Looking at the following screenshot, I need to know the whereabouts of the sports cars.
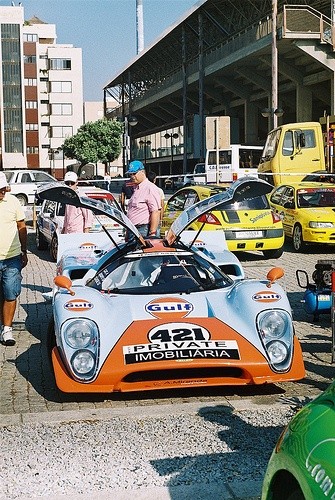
[33,177,305,393]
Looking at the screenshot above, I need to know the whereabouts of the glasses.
[65,182,75,185]
[130,172,138,175]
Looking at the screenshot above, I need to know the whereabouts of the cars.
[34,185,126,263]
[266,182,334,253]
[160,184,285,258]
[152,162,205,191]
[261,378,334,500]
[3,170,63,207]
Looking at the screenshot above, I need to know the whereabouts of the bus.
[204,145,294,184]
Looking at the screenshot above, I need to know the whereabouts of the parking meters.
[152,148,166,188]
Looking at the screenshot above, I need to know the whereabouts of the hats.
[64,171,78,183]
[125,161,144,174]
[0,171,9,189]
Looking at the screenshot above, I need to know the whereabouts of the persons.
[42,171,93,302]
[121,161,159,242]
[0,172,28,345]
[104,172,111,180]
[147,171,165,238]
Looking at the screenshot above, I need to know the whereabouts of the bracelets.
[149,231,156,235]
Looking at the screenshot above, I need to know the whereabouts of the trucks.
[258,121,335,191]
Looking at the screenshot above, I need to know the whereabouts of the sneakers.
[0,325,16,345]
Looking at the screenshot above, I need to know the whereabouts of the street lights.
[260,107,286,132]
[138,141,152,170]
[164,133,179,193]
